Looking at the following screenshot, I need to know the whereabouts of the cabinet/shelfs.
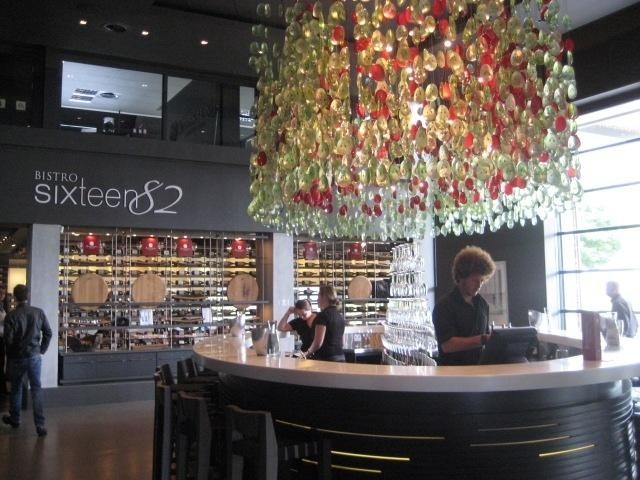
[287,233,391,335]
[59,224,272,354]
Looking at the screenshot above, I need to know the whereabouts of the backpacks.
[625,312,639,338]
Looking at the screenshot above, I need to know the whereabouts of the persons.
[431,246,496,366]
[0,285,11,395]
[606,281,638,338]
[1,284,52,437]
[277,299,319,358]
[292,285,346,362]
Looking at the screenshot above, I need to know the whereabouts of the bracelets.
[308,349,314,358]
[480,334,489,346]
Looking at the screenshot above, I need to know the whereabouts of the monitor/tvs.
[476,329,535,364]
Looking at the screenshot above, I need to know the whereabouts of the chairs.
[152,355,332,480]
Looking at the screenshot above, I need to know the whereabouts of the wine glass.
[380,241,438,367]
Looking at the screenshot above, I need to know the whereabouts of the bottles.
[294,251,393,319]
[60,246,257,346]
[608,313,619,348]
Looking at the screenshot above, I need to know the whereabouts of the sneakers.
[36,427,47,437]
[2,414,21,428]
